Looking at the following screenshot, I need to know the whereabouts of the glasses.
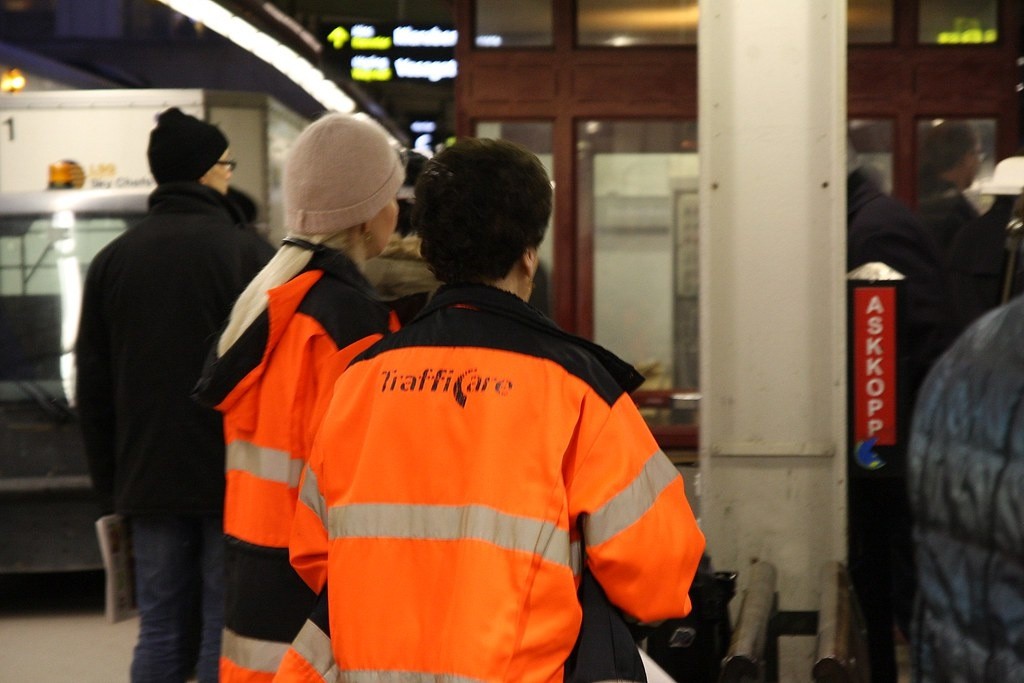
[218,159,237,178]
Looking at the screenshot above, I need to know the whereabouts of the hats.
[848,142,868,183]
[148,107,227,185]
[980,156,1024,194]
[282,114,406,234]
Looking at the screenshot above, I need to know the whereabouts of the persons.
[73,109,282,683]
[289,137,706,683]
[946,195,1024,356]
[847,138,948,683]
[895,119,979,216]
[906,295,1024,683]
[185,112,559,683]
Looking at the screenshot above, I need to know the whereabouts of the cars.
[1,185,154,574]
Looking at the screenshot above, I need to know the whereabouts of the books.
[94,513,140,623]
